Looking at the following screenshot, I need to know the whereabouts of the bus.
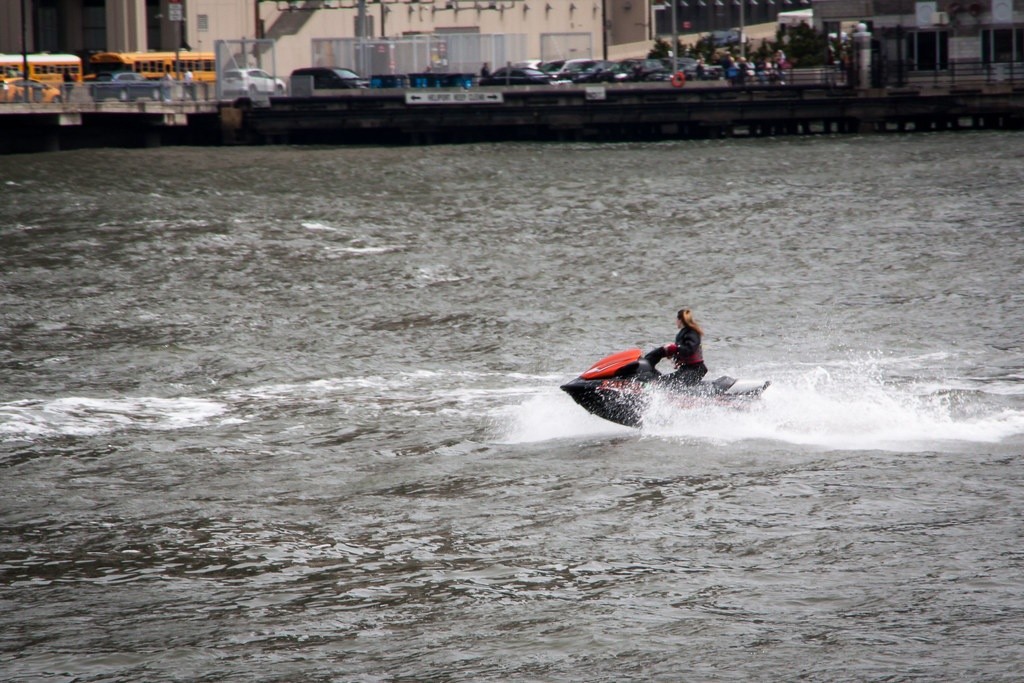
[1,53,83,94]
[87,51,218,85]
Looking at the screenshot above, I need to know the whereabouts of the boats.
[560,349,775,431]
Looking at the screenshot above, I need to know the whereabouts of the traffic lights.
[375,43,387,59]
[439,41,447,60]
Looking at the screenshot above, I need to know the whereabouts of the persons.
[6,66,17,78]
[722,50,786,85]
[481,62,490,76]
[161,71,174,101]
[181,66,196,101]
[694,52,706,81]
[63,67,75,102]
[665,308,708,387]
[828,32,851,67]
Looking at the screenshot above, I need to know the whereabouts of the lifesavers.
[671,71,686,88]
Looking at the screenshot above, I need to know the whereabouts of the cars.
[665,57,699,80]
[88,71,160,102]
[541,60,566,73]
[288,66,371,89]
[640,59,673,81]
[700,64,720,80]
[598,59,645,81]
[556,59,597,81]
[515,59,542,69]
[572,60,611,82]
[480,64,556,86]
[0,77,62,103]
[220,69,286,97]
